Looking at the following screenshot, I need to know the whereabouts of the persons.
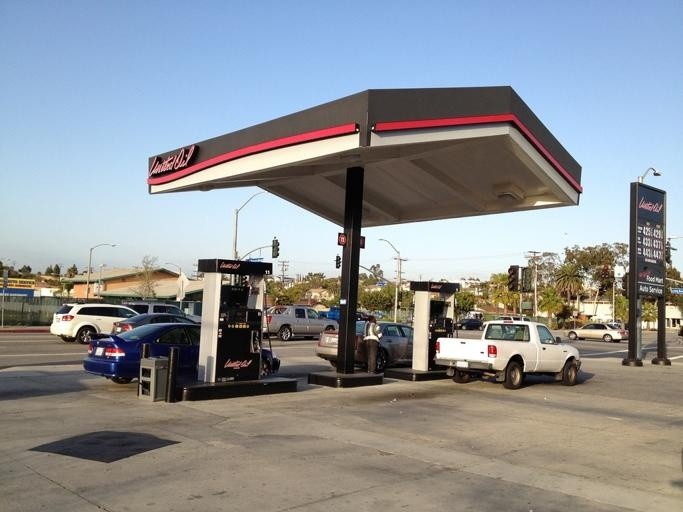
[363,315,383,373]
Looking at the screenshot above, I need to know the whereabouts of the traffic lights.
[507,266,520,293]
[335,255,341,269]
[271,238,280,258]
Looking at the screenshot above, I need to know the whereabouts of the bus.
[0,276,36,290]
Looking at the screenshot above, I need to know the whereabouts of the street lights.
[232,190,268,261]
[379,238,401,323]
[85,242,117,300]
[97,264,106,299]
[635,165,662,360]
[0,259,14,331]
[165,261,185,311]
[391,256,408,291]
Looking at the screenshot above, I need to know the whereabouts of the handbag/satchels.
[377,350,384,372]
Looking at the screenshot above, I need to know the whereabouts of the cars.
[566,321,629,344]
[46,301,281,383]
[453,311,531,331]
[261,303,415,369]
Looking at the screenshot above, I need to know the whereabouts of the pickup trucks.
[432,319,582,390]
